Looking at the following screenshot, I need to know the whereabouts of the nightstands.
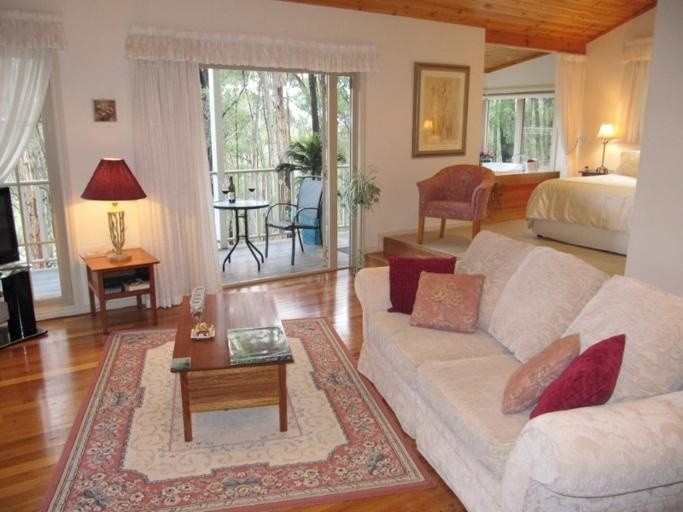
[576,167,616,178]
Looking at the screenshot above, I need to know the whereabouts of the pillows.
[406,268,487,334]
[382,251,458,318]
[498,328,584,417]
[526,332,627,420]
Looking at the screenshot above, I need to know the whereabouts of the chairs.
[412,162,497,248]
[259,174,326,267]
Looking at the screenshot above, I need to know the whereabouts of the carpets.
[34,314,439,512]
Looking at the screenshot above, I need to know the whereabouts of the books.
[225,325,292,368]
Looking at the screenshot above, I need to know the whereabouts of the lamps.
[76,153,152,263]
[592,122,618,175]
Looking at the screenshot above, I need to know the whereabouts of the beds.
[522,144,641,257]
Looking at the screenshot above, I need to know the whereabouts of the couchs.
[350,225,683,512]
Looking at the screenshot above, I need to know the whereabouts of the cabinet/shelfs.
[0,262,49,351]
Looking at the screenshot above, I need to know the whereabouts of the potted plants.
[271,130,347,247]
[342,165,382,281]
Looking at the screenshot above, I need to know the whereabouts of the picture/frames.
[409,60,473,162]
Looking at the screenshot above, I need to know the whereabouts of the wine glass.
[221,183,229,204]
[188,285,208,337]
[247,182,256,203]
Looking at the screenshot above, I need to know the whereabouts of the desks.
[212,199,270,272]
[75,245,161,338]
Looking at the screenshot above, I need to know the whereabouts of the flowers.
[476,150,496,162]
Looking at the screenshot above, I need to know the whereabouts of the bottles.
[227,176,235,203]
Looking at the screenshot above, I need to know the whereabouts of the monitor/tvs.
[0,187,20,266]
